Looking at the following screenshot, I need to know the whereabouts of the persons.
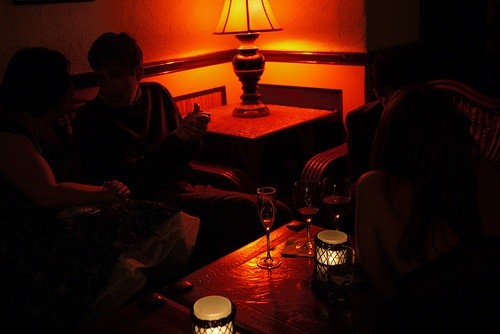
[0,32,294,334]
[346,62,500,334]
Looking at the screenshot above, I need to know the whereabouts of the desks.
[183,103,338,174]
[161,219,379,334]
[80,293,250,334]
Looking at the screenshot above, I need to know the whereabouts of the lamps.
[212,0,283,118]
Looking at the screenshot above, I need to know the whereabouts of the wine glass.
[323,177,352,232]
[295,181,320,256]
[256,188,281,268]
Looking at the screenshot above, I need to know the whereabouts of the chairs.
[301,80,500,227]
[56,96,252,195]
